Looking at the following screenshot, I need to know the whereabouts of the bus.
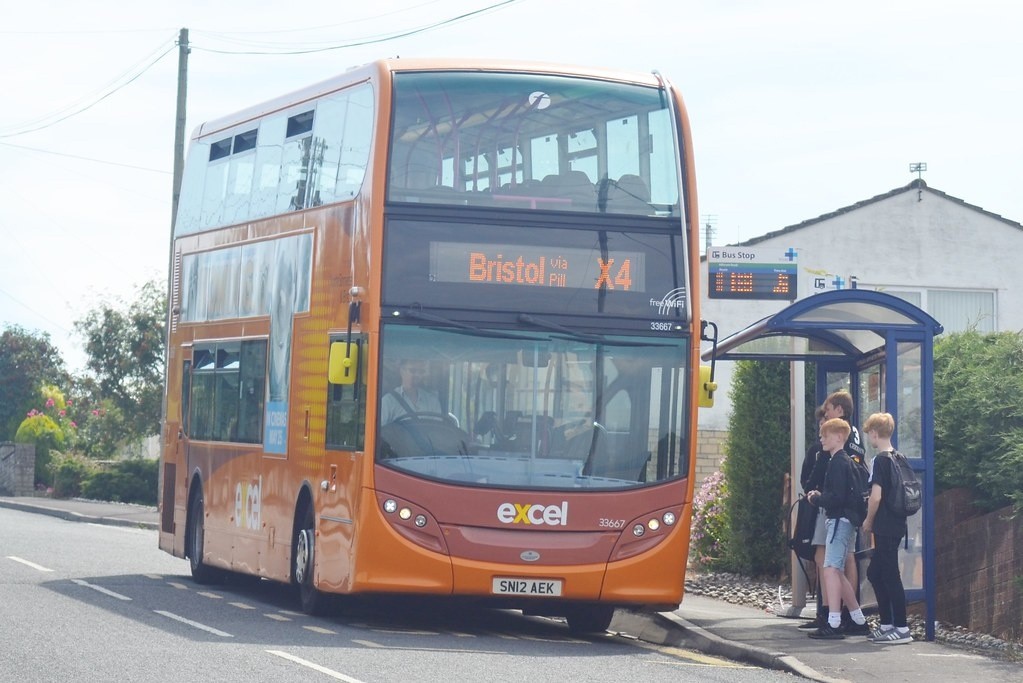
[156,56,719,637]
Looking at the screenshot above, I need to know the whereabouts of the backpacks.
[837,454,870,527]
[878,451,921,516]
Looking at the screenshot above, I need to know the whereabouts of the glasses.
[409,368,426,374]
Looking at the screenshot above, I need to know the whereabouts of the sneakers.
[867,626,894,641]
[873,627,914,644]
[842,619,869,635]
[807,622,845,639]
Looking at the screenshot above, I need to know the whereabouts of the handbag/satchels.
[794,493,818,561]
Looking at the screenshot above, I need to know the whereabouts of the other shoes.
[798,606,829,632]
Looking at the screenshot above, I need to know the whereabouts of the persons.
[381,355,442,427]
[800,390,870,639]
[864,412,914,645]
